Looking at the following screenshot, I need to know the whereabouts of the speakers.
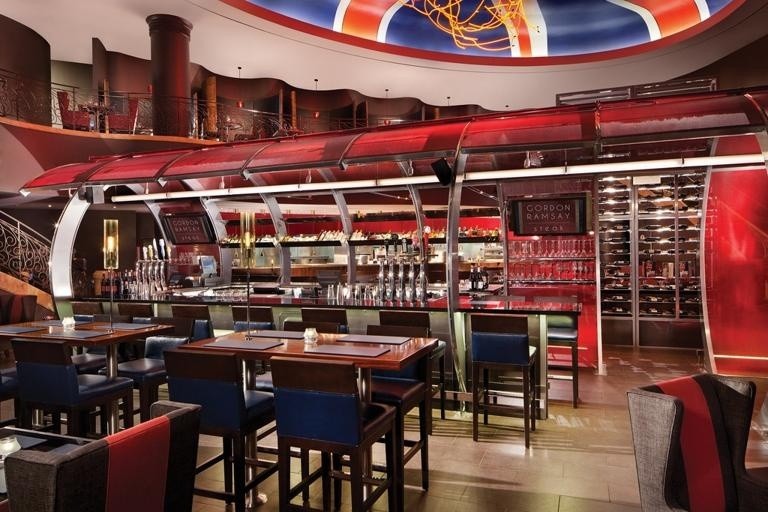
[431,158,452,186]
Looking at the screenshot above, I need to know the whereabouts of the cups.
[293,287,302,299]
[471,230,478,237]
[0,437,22,456]
[325,283,381,302]
[214,290,249,301]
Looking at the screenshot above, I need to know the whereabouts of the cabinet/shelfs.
[597,178,704,353]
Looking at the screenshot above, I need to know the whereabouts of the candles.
[244,230,252,249]
[107,235,116,252]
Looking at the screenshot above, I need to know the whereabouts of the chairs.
[57,91,91,133]
[616,372,766,510]
[470,313,538,448]
[108,96,140,135]
[212,114,306,141]
[547,312,581,409]
[1,295,446,511]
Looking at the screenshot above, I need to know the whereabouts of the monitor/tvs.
[199,256,217,274]
[160,213,217,245]
[507,197,586,236]
[169,272,186,284]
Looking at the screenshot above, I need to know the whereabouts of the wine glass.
[507,239,594,283]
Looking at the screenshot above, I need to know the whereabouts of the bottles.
[304,327,318,344]
[317,228,417,241]
[222,230,267,243]
[467,263,489,291]
[597,176,703,317]
[429,228,446,238]
[62,317,75,330]
[100,269,144,299]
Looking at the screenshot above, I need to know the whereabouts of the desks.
[78,102,115,134]
